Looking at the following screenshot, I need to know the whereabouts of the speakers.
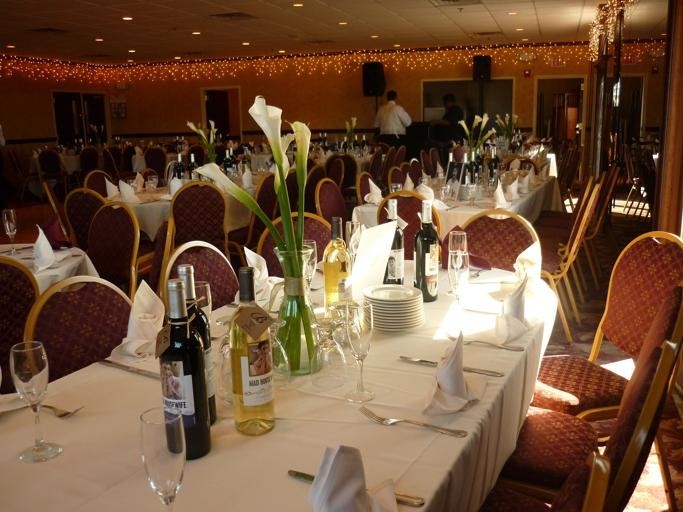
[362,62,385,96]
[472,55,491,83]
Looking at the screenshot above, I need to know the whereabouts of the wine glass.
[3,209,20,256]
[444,225,469,315]
[389,176,449,205]
[136,406,187,511]
[8,341,65,466]
[272,237,318,310]
[343,302,375,404]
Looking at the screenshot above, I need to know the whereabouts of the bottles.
[323,214,351,310]
[222,147,253,175]
[158,263,220,459]
[383,198,406,285]
[173,153,184,179]
[230,266,277,437]
[444,145,499,200]
[188,154,199,179]
[74,136,185,156]
[413,195,442,306]
[319,131,369,158]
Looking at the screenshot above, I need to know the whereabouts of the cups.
[127,175,158,197]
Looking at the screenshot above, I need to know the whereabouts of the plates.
[0,390,40,415]
[361,284,425,333]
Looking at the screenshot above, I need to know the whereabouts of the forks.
[444,335,525,352]
[360,406,467,430]
[33,399,81,419]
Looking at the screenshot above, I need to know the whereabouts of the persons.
[217,134,248,165]
[371,90,412,147]
[428,92,464,144]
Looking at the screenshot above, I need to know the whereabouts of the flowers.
[495,113,519,140]
[186,120,218,163]
[345,117,357,137]
[457,113,496,150]
[194,95,315,371]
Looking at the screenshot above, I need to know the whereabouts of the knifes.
[400,356,504,377]
[286,469,426,509]
